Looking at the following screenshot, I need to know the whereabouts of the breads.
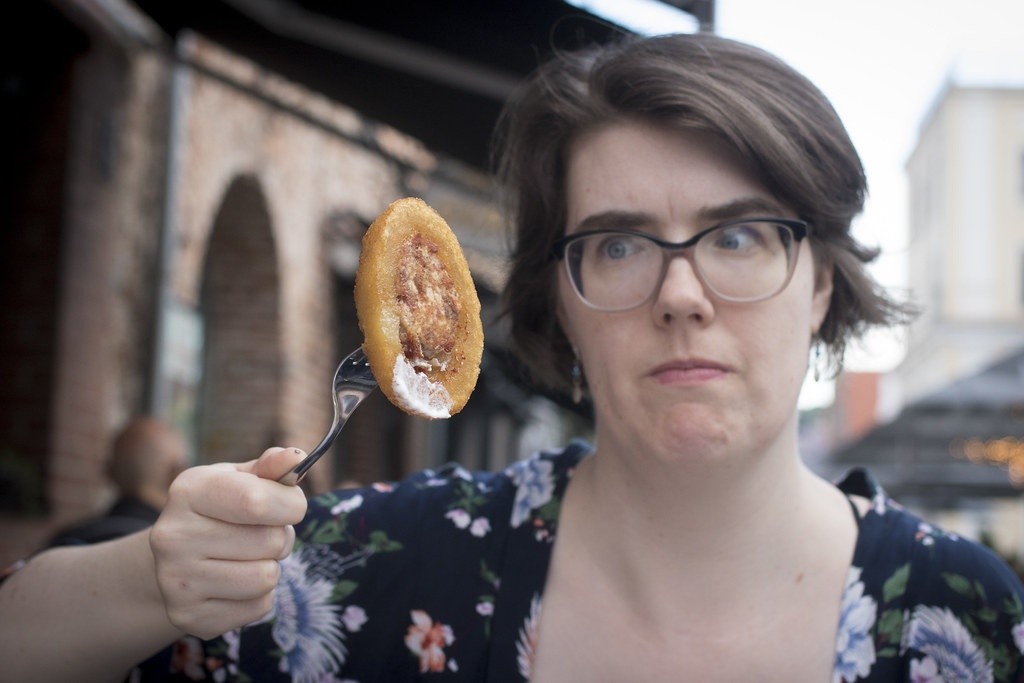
[353,197,484,419]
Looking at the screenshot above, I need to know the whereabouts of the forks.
[274,347,379,488]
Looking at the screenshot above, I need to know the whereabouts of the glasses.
[551,219,811,314]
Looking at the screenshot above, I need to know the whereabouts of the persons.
[0,32,1023,682]
[47,418,184,558]
